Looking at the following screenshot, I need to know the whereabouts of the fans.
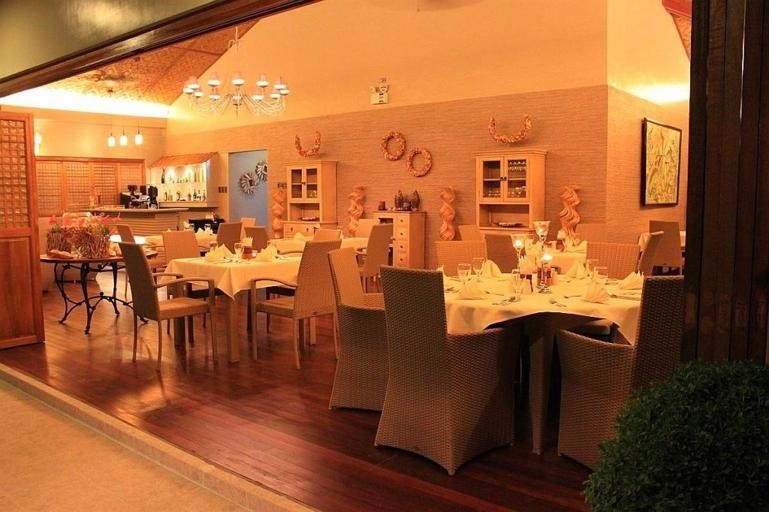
[81,75,136,91]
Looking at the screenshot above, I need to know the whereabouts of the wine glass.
[457,263,472,291]
[484,185,525,197]
[233,242,245,264]
[508,160,526,178]
[471,258,486,279]
[511,269,527,302]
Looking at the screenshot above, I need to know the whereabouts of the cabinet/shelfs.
[373,211,425,268]
[285,161,337,222]
[474,148,545,228]
[148,152,218,208]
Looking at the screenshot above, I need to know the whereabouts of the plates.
[297,217,318,221]
[493,222,522,227]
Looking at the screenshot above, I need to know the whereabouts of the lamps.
[183,26,288,118]
[103,80,116,93]
[109,56,143,148]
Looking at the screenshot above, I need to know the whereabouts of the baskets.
[73,227,110,258]
[47,228,72,254]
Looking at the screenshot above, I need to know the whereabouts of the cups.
[378,200,386,210]
[205,224,211,231]
[242,245,252,259]
[209,240,218,252]
[189,224,194,231]
[587,257,600,279]
[394,194,410,211]
[593,266,609,289]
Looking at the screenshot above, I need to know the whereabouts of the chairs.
[370,264,516,476]
[57,213,96,281]
[119,219,687,361]
[555,273,685,476]
[117,239,222,370]
[245,237,342,371]
[323,244,392,416]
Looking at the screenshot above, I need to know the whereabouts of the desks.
[39,251,158,334]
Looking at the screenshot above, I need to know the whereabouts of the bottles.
[193,188,196,199]
[161,169,165,183]
[163,190,180,201]
[203,189,205,201]
[197,189,201,201]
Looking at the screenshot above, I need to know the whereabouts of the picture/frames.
[641,118,682,207]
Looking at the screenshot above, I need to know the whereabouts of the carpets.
[0,362,323,512]
